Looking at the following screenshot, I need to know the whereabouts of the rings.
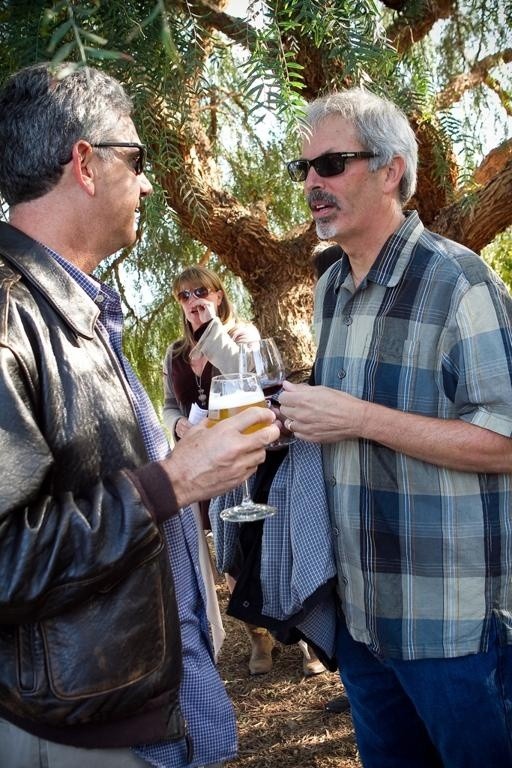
[287,420,293,431]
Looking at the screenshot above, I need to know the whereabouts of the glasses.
[177,286,213,303]
[286,150,383,182]
[59,138,148,175]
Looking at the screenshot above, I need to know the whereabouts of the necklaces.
[192,360,208,407]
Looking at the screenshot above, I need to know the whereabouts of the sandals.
[302,641,326,675]
[248,631,276,676]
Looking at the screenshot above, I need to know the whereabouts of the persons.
[160,267,328,676]
[269,89,511,768]
[1,60,283,768]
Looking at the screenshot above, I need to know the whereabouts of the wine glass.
[207,337,299,522]
